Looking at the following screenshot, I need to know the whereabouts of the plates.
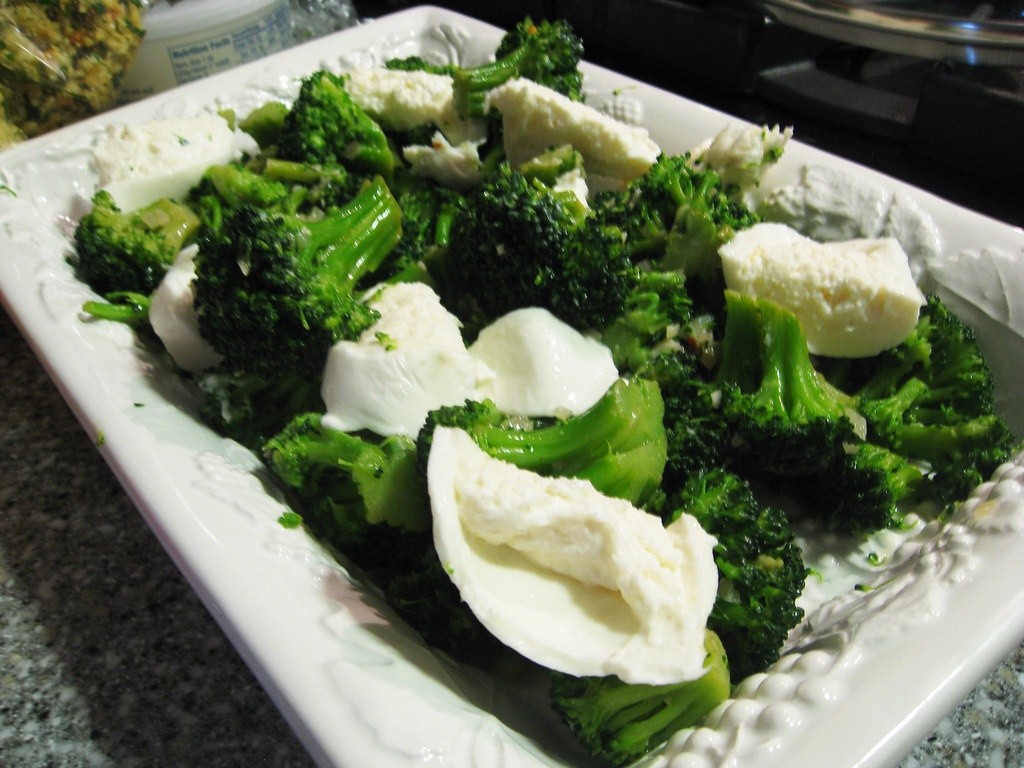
[0,0,1024,768]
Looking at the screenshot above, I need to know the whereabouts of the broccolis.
[65,20,1015,763]
